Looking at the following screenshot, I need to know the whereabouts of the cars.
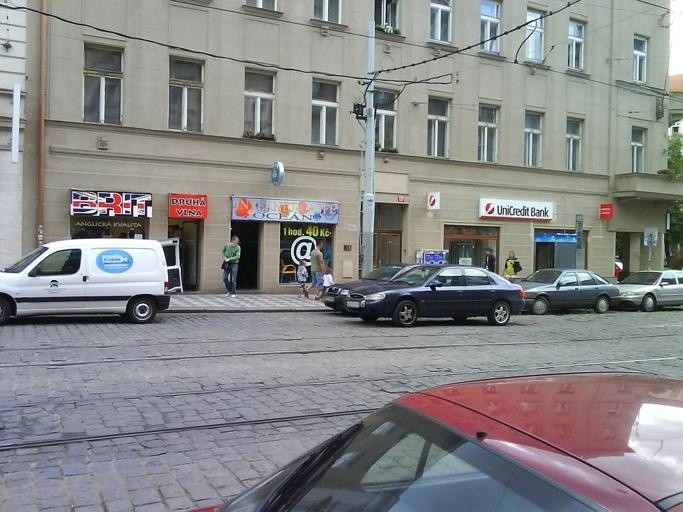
[344,265,524,327]
[614,256,624,278]
[613,270,683,312]
[512,268,624,315]
[280,246,323,270]
[176,371,683,511]
[320,263,435,317]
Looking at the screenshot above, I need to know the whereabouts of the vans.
[0,236,183,326]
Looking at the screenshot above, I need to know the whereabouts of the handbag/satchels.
[221,260,229,270]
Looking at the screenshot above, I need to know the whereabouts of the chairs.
[281,256,297,284]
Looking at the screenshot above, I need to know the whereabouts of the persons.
[222,236,241,298]
[297,260,308,297]
[484,248,495,272]
[323,267,334,293]
[503,251,517,275]
[615,266,620,280]
[303,240,328,299]
